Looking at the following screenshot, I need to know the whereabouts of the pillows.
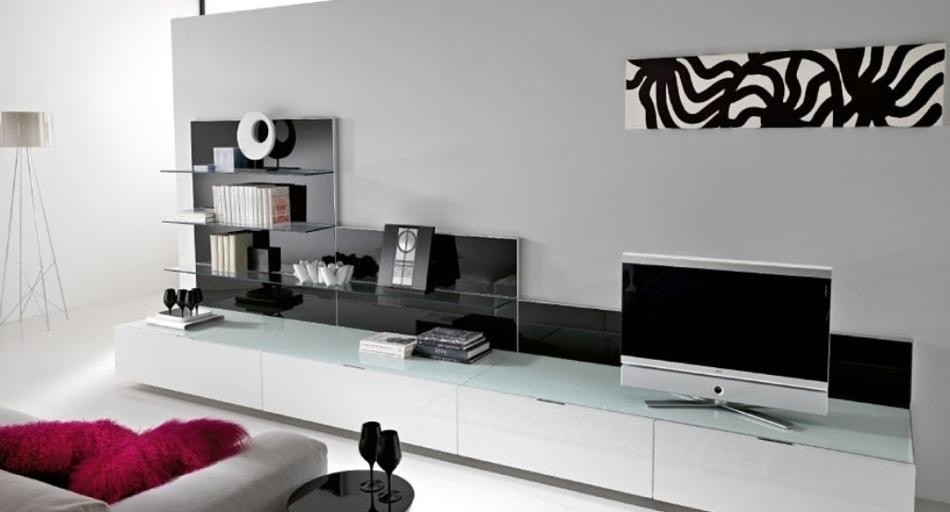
[70,418,251,506]
[0,418,138,489]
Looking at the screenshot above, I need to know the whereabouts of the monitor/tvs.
[620,250,833,430]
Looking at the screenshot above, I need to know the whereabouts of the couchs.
[0,407,328,512]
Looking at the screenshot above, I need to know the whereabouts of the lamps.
[0,112,70,331]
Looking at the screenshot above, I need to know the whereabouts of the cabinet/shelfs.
[160,119,519,349]
[114,305,916,512]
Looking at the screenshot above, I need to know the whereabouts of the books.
[212,182,292,230]
[358,331,419,360]
[412,326,493,364]
[209,230,254,277]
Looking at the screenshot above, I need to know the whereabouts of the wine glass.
[185,290,195,316]
[174,289,187,316]
[377,429,403,503]
[191,287,203,316]
[367,492,378,511]
[164,288,176,315]
[357,420,385,492]
